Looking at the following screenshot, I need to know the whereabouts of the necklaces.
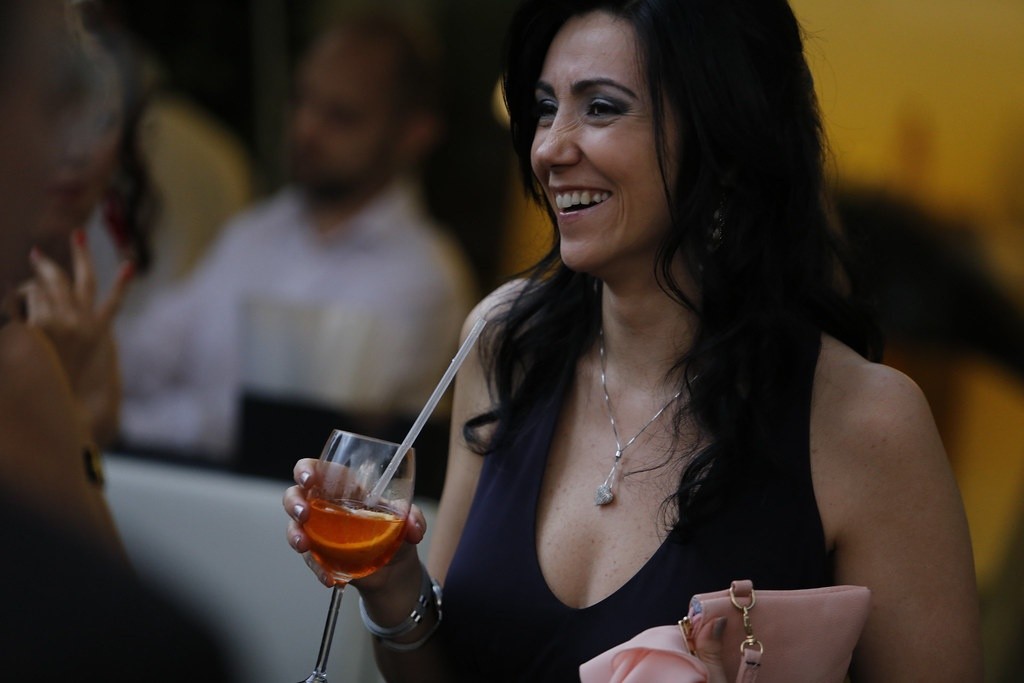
[595,328,698,506]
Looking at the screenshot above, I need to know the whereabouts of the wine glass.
[293,429,418,683]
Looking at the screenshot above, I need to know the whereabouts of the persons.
[285,0,980,683]
[113,21,471,467]
[0,0,231,683]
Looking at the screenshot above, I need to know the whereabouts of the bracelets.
[378,577,441,652]
[358,561,431,639]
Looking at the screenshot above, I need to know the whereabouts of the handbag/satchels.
[677,579,871,683]
